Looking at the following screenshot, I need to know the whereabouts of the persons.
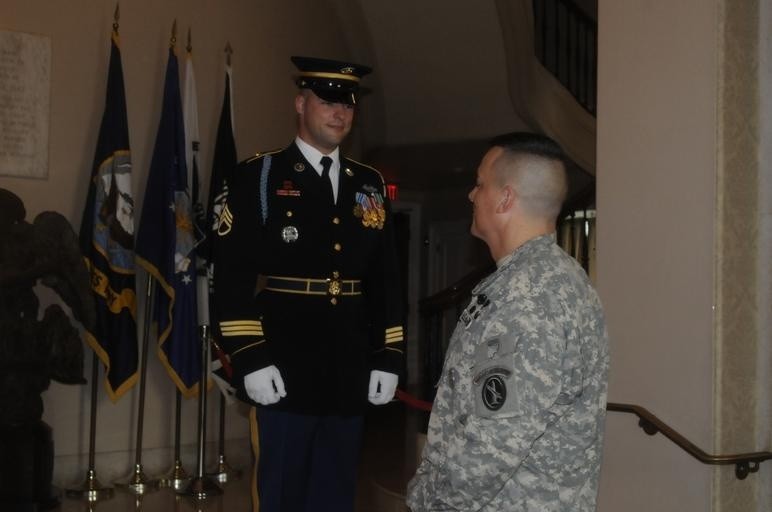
[209,54,408,512]
[404,130,613,510]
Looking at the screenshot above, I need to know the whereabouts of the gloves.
[243,364,288,406]
[367,369,399,406]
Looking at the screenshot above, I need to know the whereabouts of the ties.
[320,156,335,209]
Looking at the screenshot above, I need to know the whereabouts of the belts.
[263,275,362,296]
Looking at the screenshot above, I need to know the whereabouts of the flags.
[77,21,237,404]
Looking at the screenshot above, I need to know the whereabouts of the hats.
[290,55,374,106]
